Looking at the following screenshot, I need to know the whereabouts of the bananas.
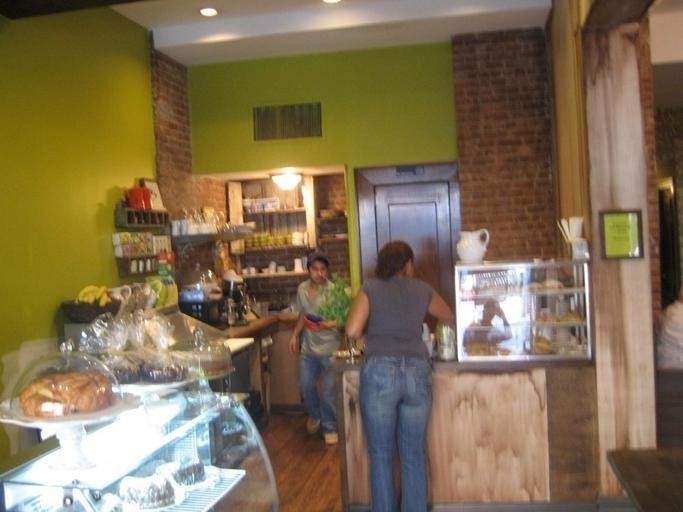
[74,284,112,308]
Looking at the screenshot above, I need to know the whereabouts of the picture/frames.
[598,209,643,260]
[138,177,164,208]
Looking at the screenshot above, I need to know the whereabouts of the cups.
[177,206,239,233]
[237,256,307,274]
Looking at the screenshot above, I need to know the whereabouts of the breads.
[19,370,113,417]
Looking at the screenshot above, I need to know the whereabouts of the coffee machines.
[221,270,249,327]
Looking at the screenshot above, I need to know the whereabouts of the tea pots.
[457,228,489,266]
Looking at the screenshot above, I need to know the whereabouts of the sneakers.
[325,432,338,444]
[305,417,320,433]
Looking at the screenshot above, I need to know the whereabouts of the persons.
[286,252,355,446]
[655,281,683,377]
[462,299,511,353]
[343,238,454,511]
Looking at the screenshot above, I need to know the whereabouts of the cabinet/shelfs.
[454,259,596,363]
[0,390,280,510]
[227,176,316,280]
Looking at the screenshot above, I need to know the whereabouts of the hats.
[307,254,328,267]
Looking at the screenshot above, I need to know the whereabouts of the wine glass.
[344,336,358,364]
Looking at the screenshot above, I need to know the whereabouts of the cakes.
[157,459,205,486]
[117,474,174,509]
